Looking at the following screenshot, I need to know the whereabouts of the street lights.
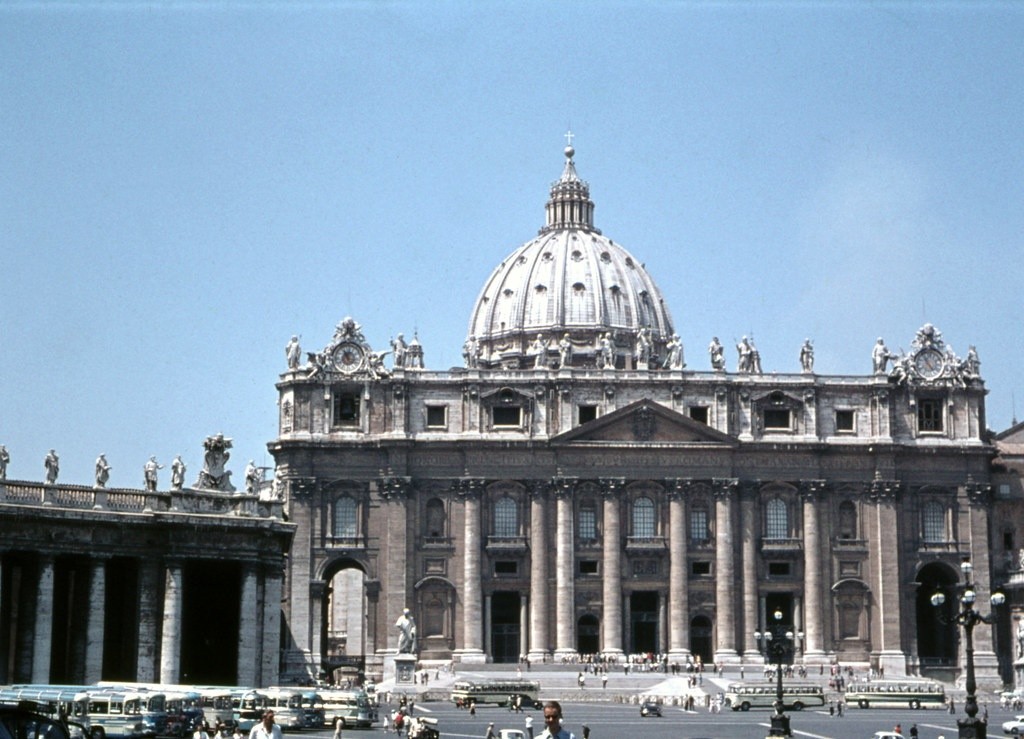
[931,556,1006,739]
[754,611,805,739]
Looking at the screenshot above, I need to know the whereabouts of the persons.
[535,702,576,739]
[599,332,617,368]
[394,608,417,653]
[271,466,288,498]
[666,333,684,369]
[0,650,1023,739]
[559,333,572,366]
[172,454,188,489]
[249,709,282,739]
[44,449,59,484]
[637,328,652,362]
[736,335,752,371]
[892,344,979,389]
[532,333,547,367]
[285,335,301,369]
[246,459,260,493]
[465,335,480,369]
[871,337,889,372]
[708,336,725,369]
[800,338,814,371]
[391,333,408,365]
[95,453,111,487]
[0,444,10,480]
[145,454,164,491]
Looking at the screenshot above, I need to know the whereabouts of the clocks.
[914,348,945,380]
[332,341,365,375]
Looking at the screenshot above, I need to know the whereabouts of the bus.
[844,680,949,710]
[0,681,379,739]
[724,683,825,712]
[450,679,540,708]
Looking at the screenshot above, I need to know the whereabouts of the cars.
[640,702,662,718]
[496,729,525,739]
[872,731,905,739]
[1002,715,1024,735]
